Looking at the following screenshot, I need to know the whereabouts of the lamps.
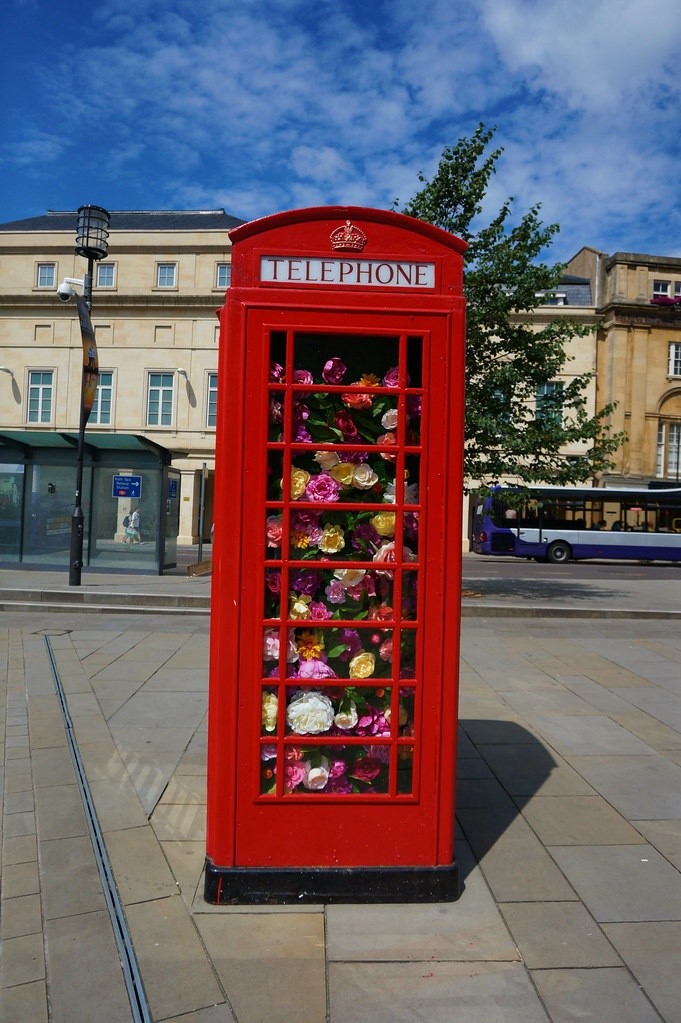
[0,366,14,375]
[177,367,187,379]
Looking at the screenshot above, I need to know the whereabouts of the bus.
[469,485,681,564]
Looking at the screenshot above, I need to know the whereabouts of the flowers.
[262,355,413,797]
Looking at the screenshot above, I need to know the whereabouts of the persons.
[129,507,145,545]
[576,517,649,532]
[121,509,139,545]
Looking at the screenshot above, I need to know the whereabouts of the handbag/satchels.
[124,528,136,539]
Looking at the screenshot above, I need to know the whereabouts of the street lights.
[57,204,110,585]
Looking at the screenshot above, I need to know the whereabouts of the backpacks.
[122,515,130,528]
